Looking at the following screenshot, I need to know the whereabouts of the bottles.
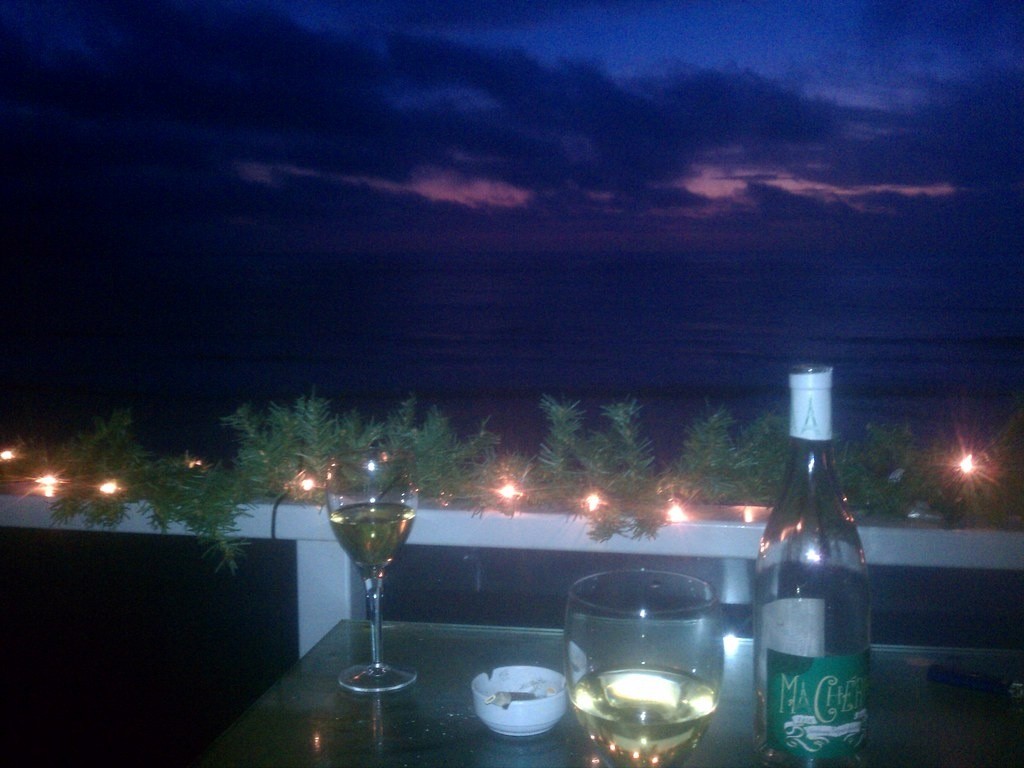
[754,364,874,768]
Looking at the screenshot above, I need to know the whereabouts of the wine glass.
[325,447,419,694]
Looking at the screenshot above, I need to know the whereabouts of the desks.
[204,619,1024,767]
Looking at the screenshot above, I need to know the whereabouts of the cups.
[563,569,725,768]
